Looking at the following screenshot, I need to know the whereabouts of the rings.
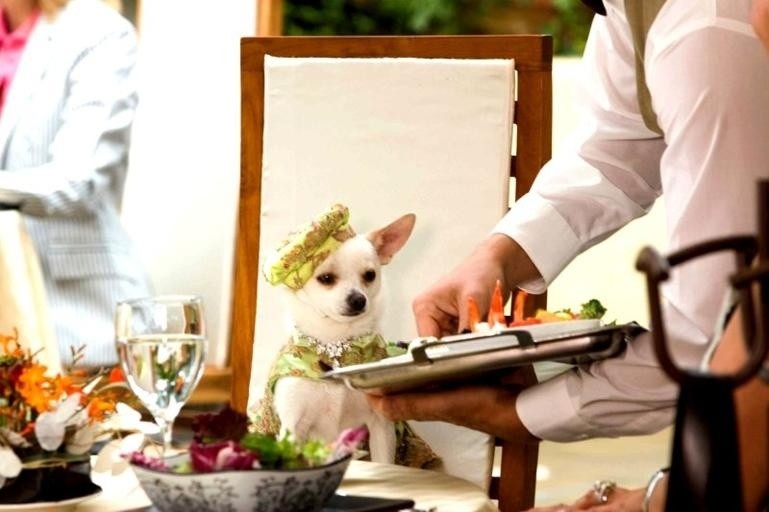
[591,479,616,504]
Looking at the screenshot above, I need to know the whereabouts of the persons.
[521,257,769,512]
[1,1,143,378]
[364,1,768,445]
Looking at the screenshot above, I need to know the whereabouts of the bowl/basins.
[129,451,353,512]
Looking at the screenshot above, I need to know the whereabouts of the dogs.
[261,203,447,475]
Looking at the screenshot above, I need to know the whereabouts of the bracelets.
[638,464,670,512]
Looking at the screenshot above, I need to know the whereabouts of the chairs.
[224,33,557,512]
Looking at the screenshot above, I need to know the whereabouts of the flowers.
[0,329,163,478]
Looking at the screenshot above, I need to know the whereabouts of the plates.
[0,455,152,512]
[440,317,601,356]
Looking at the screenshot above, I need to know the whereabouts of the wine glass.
[115,295,207,458]
